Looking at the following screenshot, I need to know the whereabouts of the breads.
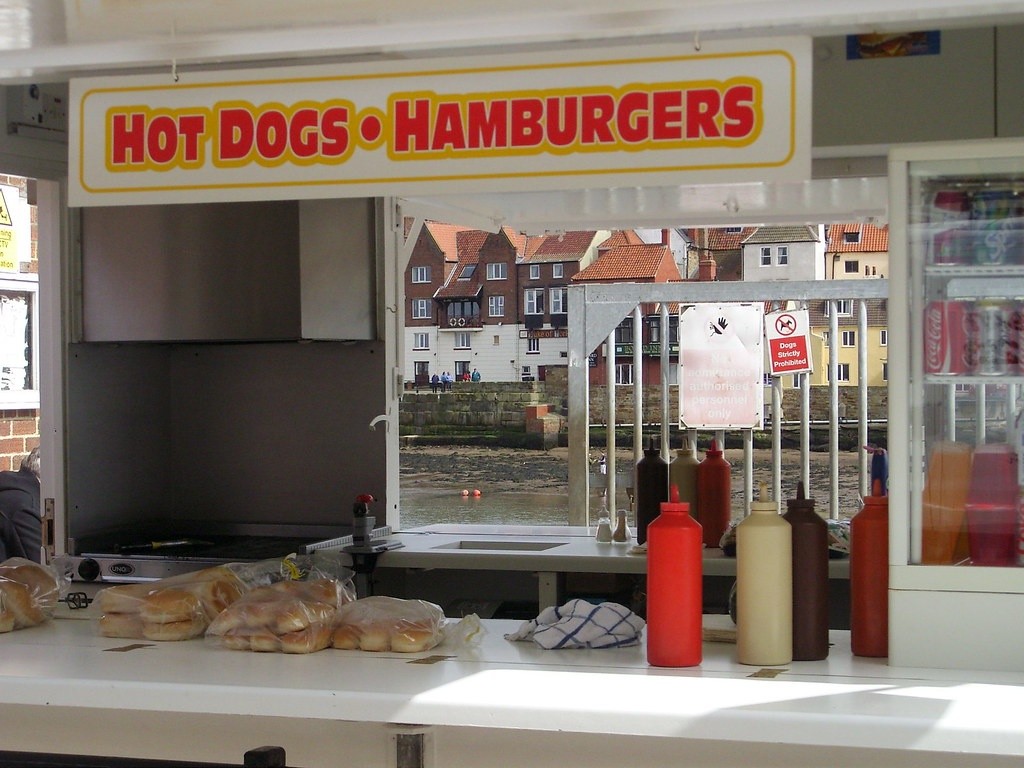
[92,565,444,653]
[0,563,61,633]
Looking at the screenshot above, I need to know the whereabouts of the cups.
[923,441,969,566]
[965,445,1014,567]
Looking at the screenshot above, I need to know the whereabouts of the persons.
[464,371,471,382]
[440,372,447,392]
[472,369,481,382]
[1,445,40,562]
[432,373,439,393]
[446,372,454,390]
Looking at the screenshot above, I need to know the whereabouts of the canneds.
[924,296,1024,376]
[930,186,1024,266]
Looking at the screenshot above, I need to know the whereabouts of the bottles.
[849,479,888,657]
[782,481,828,661]
[669,439,701,522]
[611,511,632,543]
[698,439,731,548]
[596,505,612,543]
[646,482,702,667]
[736,482,793,665]
[637,438,669,545]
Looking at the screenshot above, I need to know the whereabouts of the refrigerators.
[887,138,1024,672]
